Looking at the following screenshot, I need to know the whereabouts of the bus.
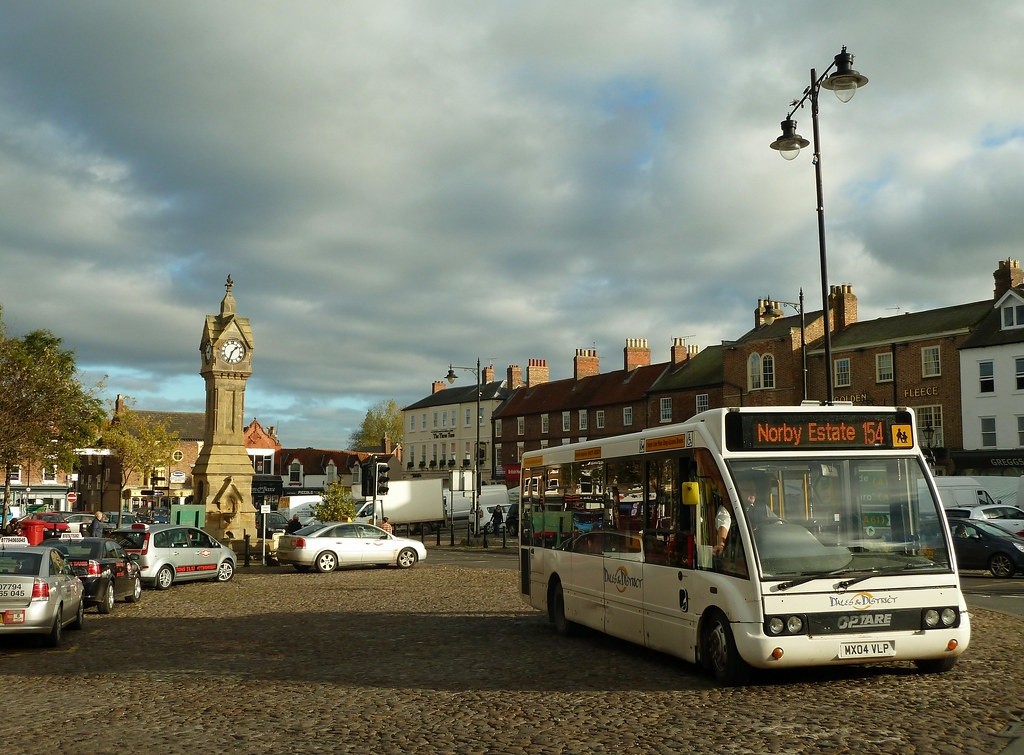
[518,404,970,685]
[524,495,615,540]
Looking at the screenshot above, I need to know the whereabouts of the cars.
[20,532,142,614]
[882,518,1024,578]
[277,521,428,573]
[0,540,85,643]
[14,510,136,538]
[256,511,290,538]
[505,503,518,535]
[108,523,238,590]
[934,503,1024,538]
[469,502,515,533]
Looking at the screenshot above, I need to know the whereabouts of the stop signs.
[67,492,77,503]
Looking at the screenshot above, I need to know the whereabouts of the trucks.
[0,498,51,535]
[353,478,444,536]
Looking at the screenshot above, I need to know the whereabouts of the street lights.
[761,300,808,400]
[769,43,869,407]
[442,365,480,537]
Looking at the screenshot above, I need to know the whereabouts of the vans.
[554,530,645,562]
[278,495,327,523]
[443,484,511,520]
[917,477,1002,519]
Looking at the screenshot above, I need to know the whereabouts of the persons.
[712,478,782,558]
[284,514,302,535]
[954,525,970,538]
[380,517,392,535]
[469,502,504,537]
[89,511,103,559]
[5,518,22,536]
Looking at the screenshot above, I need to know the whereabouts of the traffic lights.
[376,463,389,495]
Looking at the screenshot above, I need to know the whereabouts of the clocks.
[219,338,246,365]
[202,342,212,363]
